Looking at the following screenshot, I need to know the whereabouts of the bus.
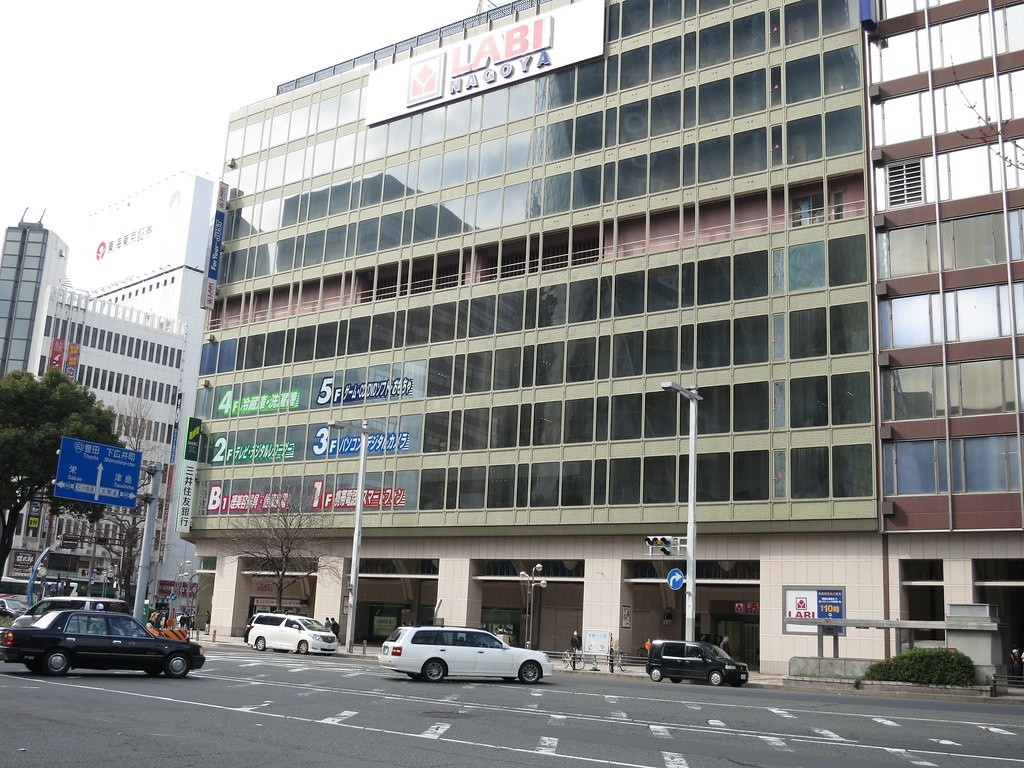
[0,576,79,619]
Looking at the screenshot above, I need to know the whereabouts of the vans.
[10,595,133,629]
[645,638,749,686]
[244,612,339,656]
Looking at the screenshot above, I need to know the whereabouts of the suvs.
[377,624,556,684]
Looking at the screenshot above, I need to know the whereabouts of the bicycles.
[636,646,647,658]
[607,647,628,674]
[561,650,586,670]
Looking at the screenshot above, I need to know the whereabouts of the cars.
[0,610,206,680]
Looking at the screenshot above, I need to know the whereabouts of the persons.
[1010,644,1024,688]
[571,630,582,663]
[325,617,331,628]
[149,608,176,630]
[497,624,513,646]
[331,618,342,646]
[204,611,211,635]
[720,634,731,657]
[180,612,191,631]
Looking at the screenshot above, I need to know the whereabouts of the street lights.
[325,415,370,652]
[657,376,703,641]
[518,563,548,649]
[172,558,192,612]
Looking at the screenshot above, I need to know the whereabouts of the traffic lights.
[82,570,86,576]
[645,536,673,547]
